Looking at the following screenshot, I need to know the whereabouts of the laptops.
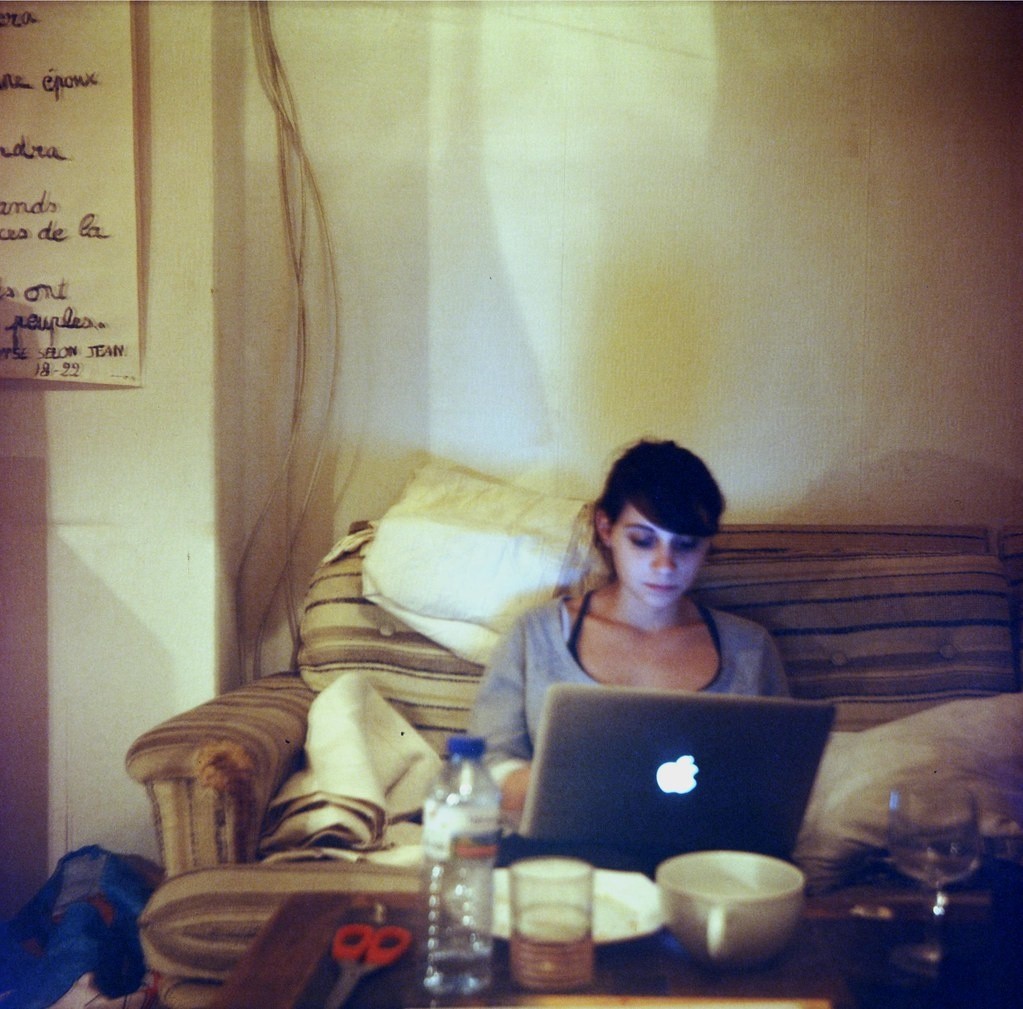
[488,681,836,869]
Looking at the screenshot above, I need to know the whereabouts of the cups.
[656,845,805,967]
[506,853,596,991]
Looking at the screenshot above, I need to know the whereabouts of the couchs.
[123,475,1021,1009]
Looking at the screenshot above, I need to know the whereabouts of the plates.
[447,862,670,942]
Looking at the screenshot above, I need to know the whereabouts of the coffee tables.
[206,882,1021,1004]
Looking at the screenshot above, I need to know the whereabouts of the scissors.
[323,923,412,1009]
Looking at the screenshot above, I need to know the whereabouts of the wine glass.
[884,779,988,980]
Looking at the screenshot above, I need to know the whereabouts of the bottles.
[412,730,503,995]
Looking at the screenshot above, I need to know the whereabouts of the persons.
[465,440,793,810]
